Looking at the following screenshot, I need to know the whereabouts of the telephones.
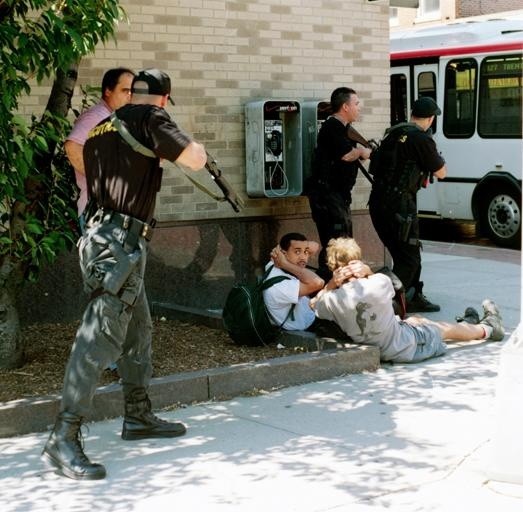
[264,122,286,189]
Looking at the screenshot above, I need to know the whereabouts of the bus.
[390,6,523,252]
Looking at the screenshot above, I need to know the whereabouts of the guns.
[205,150,246,213]
[420,128,434,188]
[394,213,413,242]
[347,124,378,161]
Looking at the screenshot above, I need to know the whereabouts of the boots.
[40,414,105,479]
[122,398,186,440]
[406,286,439,312]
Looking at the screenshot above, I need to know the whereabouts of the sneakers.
[457,307,479,323]
[480,299,505,341]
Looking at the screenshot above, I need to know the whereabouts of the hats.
[413,97,441,116]
[131,70,175,105]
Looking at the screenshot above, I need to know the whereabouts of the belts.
[111,213,154,240]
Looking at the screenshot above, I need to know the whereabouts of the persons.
[310,87,372,282]
[261,233,415,337]
[40,69,207,480]
[64,68,155,378]
[308,236,504,364]
[366,96,446,313]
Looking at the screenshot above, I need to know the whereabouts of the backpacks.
[223,264,295,346]
